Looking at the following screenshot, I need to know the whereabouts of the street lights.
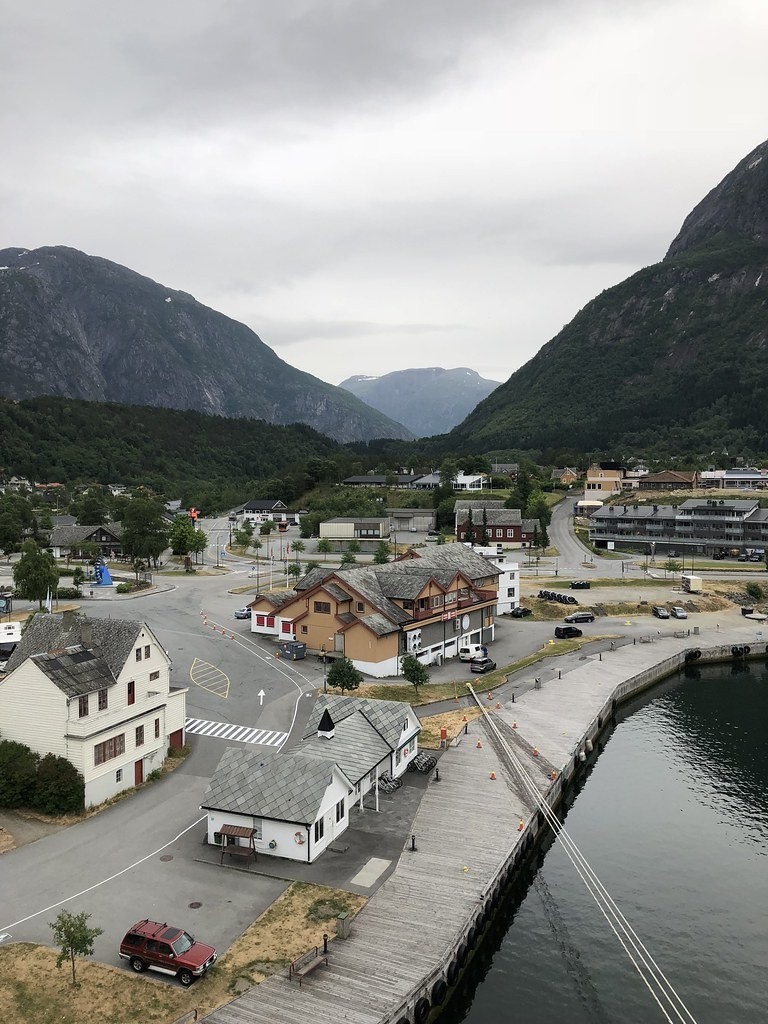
[319,643,327,694]
[228,523,231,547]
[257,532,298,591]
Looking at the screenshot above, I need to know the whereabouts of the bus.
[278,521,290,532]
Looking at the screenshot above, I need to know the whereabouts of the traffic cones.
[512,719,519,728]
[476,737,482,748]
[489,768,496,780]
[533,745,539,757]
[487,690,493,700]
[454,693,458,703]
[199,608,235,640]
[517,817,524,831]
[462,710,468,721]
[551,768,557,780]
[495,700,501,709]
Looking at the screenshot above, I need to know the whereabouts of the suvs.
[118,918,218,985]
[555,625,583,638]
[471,656,496,674]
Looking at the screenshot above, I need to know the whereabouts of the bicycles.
[407,750,437,774]
[372,769,403,794]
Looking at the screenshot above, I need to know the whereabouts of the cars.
[652,606,670,619]
[390,523,395,532]
[644,548,656,555]
[248,521,257,529]
[713,552,762,561]
[670,606,687,619]
[425,536,438,542]
[428,530,441,536]
[668,551,680,557]
[235,608,251,619]
[290,521,298,526]
[228,511,237,521]
[278,639,307,661]
[511,606,532,618]
[411,527,417,533]
[564,611,595,623]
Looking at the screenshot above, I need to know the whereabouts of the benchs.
[289,946,328,986]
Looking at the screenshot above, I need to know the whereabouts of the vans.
[459,643,488,662]
